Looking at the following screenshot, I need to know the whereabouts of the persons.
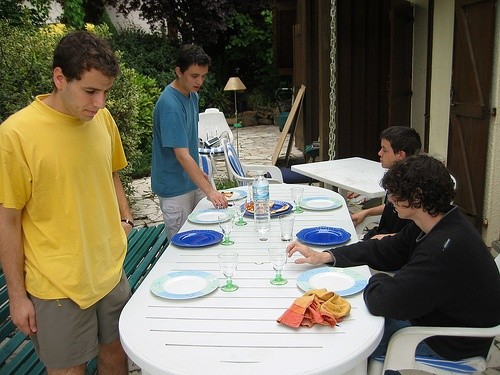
[149,43,228,243]
[285,155,500,359]
[350,125,422,241]
[0,29,135,375]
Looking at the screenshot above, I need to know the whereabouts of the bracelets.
[121,219,134,229]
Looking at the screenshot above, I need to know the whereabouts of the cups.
[278,214,295,242]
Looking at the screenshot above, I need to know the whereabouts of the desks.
[289,155,389,200]
[117,181,385,375]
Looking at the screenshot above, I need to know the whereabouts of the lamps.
[224,76,247,158]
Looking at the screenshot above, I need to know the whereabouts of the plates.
[240,200,293,217]
[297,266,369,297]
[217,189,248,200]
[150,271,220,299]
[170,230,223,248]
[296,226,351,246]
[188,207,234,224]
[299,196,343,211]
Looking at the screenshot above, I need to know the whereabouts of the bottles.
[252,170,271,232]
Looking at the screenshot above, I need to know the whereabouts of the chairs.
[353,170,458,241]
[198,109,234,183]
[220,137,284,185]
[367,253,500,375]
[198,153,217,190]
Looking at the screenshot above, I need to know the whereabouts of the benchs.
[0,221,168,375]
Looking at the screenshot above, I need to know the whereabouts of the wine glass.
[233,198,247,226]
[291,187,304,214]
[218,250,239,292]
[269,245,287,285]
[219,214,235,246]
